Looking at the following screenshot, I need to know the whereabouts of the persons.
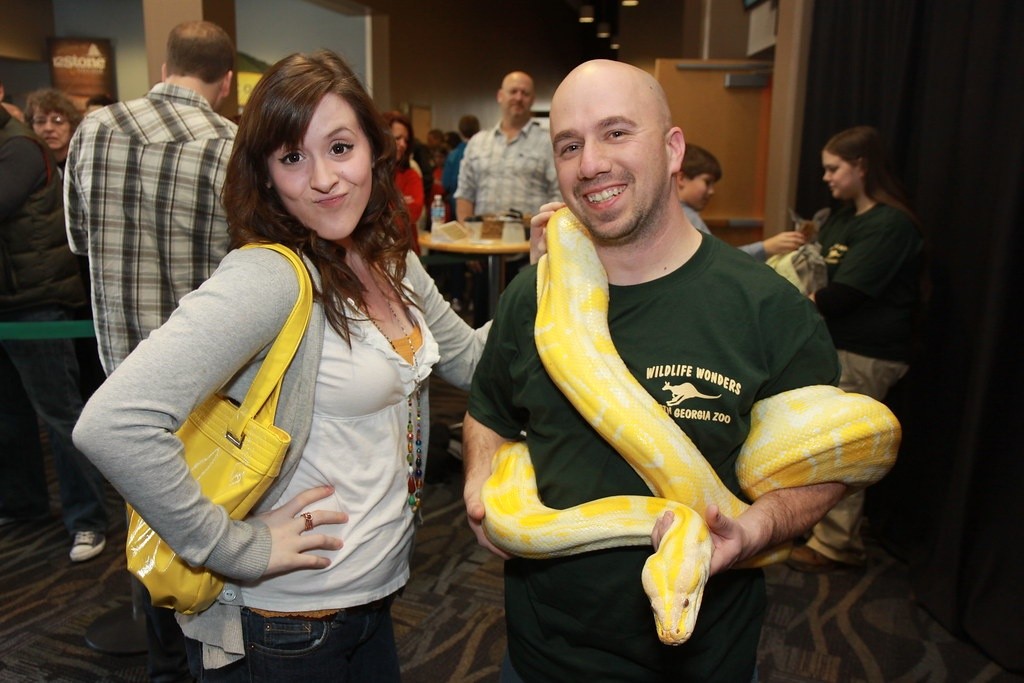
[791,124,926,573]
[381,110,486,313]
[674,144,806,263]
[464,58,845,683]
[1,82,116,563]
[453,71,561,222]
[26,87,80,186]
[59,20,241,386]
[70,47,567,683]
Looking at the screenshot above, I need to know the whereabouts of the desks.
[416,223,555,470]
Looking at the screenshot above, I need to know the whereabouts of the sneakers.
[69,529,106,562]
[787,543,845,573]
[0,507,52,526]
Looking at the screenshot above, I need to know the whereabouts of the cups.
[464,215,484,244]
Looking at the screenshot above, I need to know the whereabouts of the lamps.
[580,0,640,50]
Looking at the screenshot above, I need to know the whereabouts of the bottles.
[430,194,446,238]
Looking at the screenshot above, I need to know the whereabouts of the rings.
[301,512,313,529]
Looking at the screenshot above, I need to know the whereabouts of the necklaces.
[354,266,424,512]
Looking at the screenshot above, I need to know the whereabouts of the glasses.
[30,114,69,125]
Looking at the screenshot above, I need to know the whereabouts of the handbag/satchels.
[126,242,313,614]
